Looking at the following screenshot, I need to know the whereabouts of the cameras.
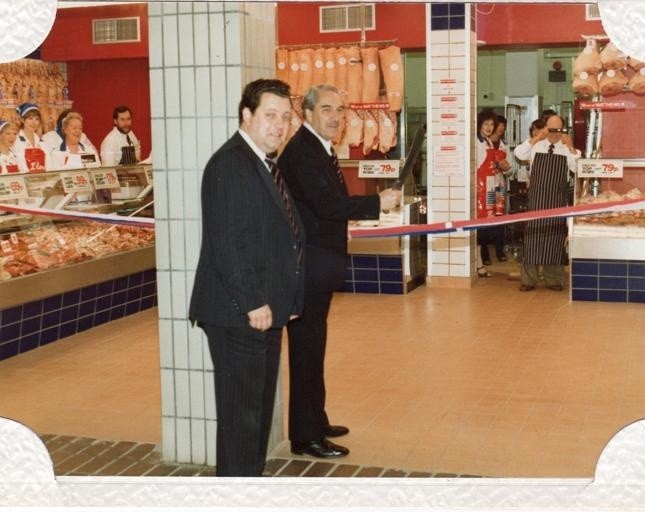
[549,128,567,133]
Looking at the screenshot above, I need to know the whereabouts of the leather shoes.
[324,425,350,438]
[547,285,563,291]
[519,284,535,292]
[288,434,350,459]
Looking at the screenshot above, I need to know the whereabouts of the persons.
[0,103,141,205]
[477,109,582,292]
[189,78,307,476]
[277,84,403,460]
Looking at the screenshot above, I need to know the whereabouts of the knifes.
[382,127,424,216]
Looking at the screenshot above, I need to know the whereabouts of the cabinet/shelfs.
[0,162,155,312]
[565,157,645,260]
[337,160,426,255]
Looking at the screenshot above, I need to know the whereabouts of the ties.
[330,146,344,188]
[548,144,554,154]
[264,158,302,273]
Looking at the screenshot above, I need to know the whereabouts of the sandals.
[477,250,507,278]
